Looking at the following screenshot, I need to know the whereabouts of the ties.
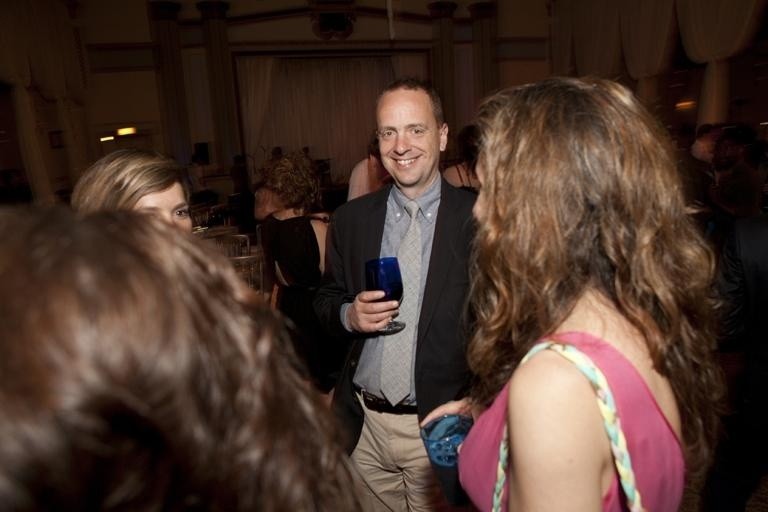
[378,201,423,407]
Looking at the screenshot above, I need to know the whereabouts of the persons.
[0,68,768,511]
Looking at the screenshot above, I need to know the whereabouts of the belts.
[354,385,419,416]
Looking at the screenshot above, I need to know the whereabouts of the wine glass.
[365,256,406,334]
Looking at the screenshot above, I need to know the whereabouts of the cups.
[420,414,474,483]
